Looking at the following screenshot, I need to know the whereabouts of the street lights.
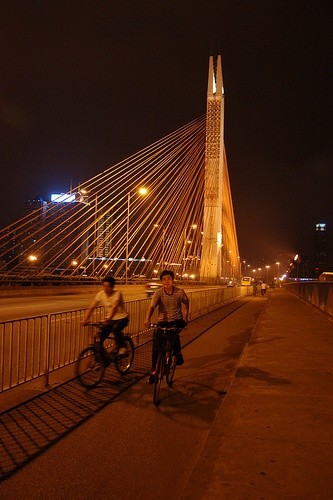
[80,189,98,277]
[153,224,165,273]
[242,260,279,281]
[125,186,148,284]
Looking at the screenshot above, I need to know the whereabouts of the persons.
[253,281,258,295]
[79,276,129,371]
[260,281,267,296]
[142,270,190,384]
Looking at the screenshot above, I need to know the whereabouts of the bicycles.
[146,322,186,406]
[75,320,136,389]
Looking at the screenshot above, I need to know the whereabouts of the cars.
[144,278,165,298]
[227,281,236,288]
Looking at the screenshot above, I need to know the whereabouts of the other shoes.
[175,354,184,365]
[93,362,102,370]
[117,347,128,355]
[147,374,156,383]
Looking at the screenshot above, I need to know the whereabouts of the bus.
[241,277,252,286]
